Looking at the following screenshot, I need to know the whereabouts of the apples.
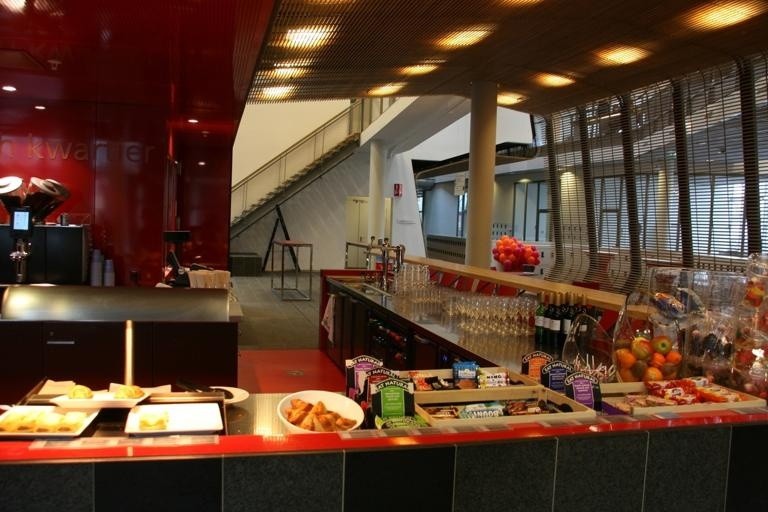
[631,336,650,359]
[652,336,672,355]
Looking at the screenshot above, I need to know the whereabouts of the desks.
[270,240,313,302]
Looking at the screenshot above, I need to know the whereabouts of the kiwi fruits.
[631,359,648,377]
[663,364,677,380]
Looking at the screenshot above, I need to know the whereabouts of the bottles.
[103,260,116,287]
[538,291,591,359]
[91,249,102,287]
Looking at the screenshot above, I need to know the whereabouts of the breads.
[66,383,95,401]
[286,399,356,432]
[107,381,147,399]
[0,410,88,435]
[138,410,170,428]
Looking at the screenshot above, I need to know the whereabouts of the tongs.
[170,373,234,400]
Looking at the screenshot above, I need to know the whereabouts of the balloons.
[492,235,541,272]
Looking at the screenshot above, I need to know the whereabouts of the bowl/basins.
[276,389,365,433]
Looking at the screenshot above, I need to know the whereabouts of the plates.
[185,385,250,405]
[0,404,101,438]
[123,404,224,436]
[48,390,154,409]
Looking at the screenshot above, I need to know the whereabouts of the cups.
[188,268,231,288]
[394,289,455,320]
[394,263,430,290]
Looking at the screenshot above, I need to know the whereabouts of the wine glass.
[455,292,538,338]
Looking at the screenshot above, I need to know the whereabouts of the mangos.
[620,368,640,382]
